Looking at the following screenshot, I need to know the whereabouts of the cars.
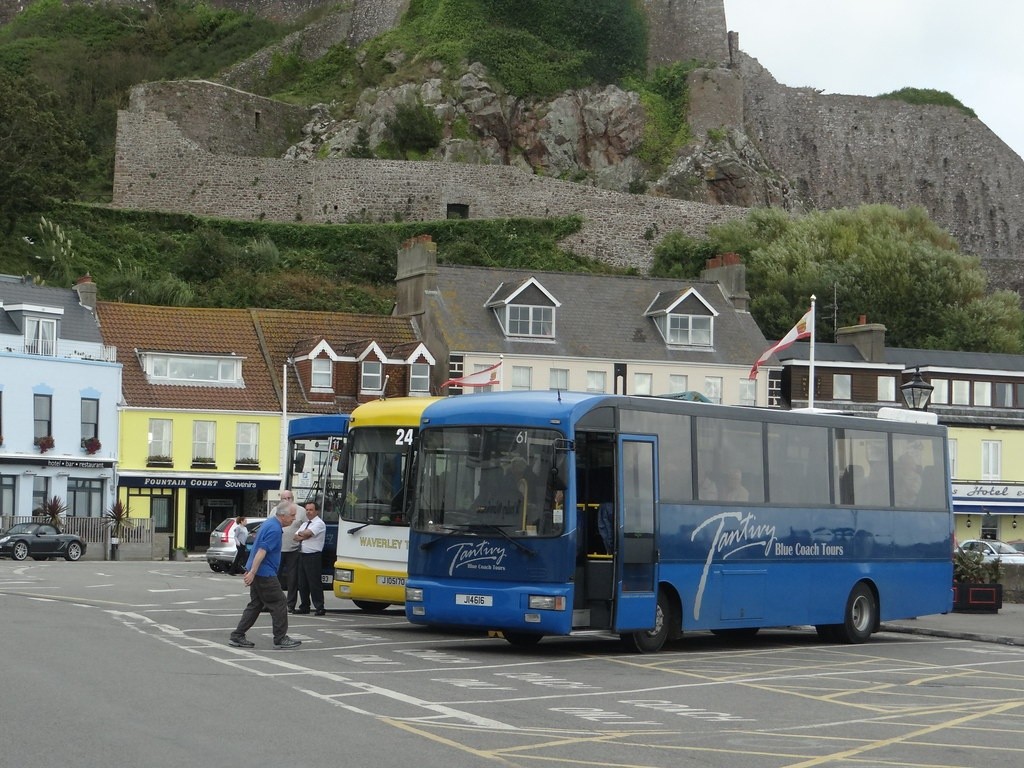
[0,523,88,561]
[205,515,267,576]
[954,539,1024,566]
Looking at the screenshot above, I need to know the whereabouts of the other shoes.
[228,572,235,575]
[314,609,326,615]
[287,608,294,615]
[260,607,270,612]
[241,571,245,574]
[292,608,310,614]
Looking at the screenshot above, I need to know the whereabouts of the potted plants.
[172,546,188,562]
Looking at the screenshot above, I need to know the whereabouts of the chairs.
[922,465,945,508]
[868,472,887,497]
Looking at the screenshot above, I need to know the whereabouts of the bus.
[282,413,350,593]
[334,394,453,615]
[403,386,956,656]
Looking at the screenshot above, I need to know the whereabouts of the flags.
[440,362,502,386]
[748,309,812,380]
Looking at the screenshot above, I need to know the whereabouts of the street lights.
[900,364,934,410]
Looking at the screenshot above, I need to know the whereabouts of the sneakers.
[273,635,301,647]
[228,634,255,647]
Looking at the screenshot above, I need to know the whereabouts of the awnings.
[116,472,281,490]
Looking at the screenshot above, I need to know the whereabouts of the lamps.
[966,514,971,528]
[1012,515,1017,529]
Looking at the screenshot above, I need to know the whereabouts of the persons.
[229,516,252,576]
[697,462,749,501]
[293,503,327,616]
[261,490,308,613]
[228,501,302,649]
[894,453,922,507]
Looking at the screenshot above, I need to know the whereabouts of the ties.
[297,521,312,552]
